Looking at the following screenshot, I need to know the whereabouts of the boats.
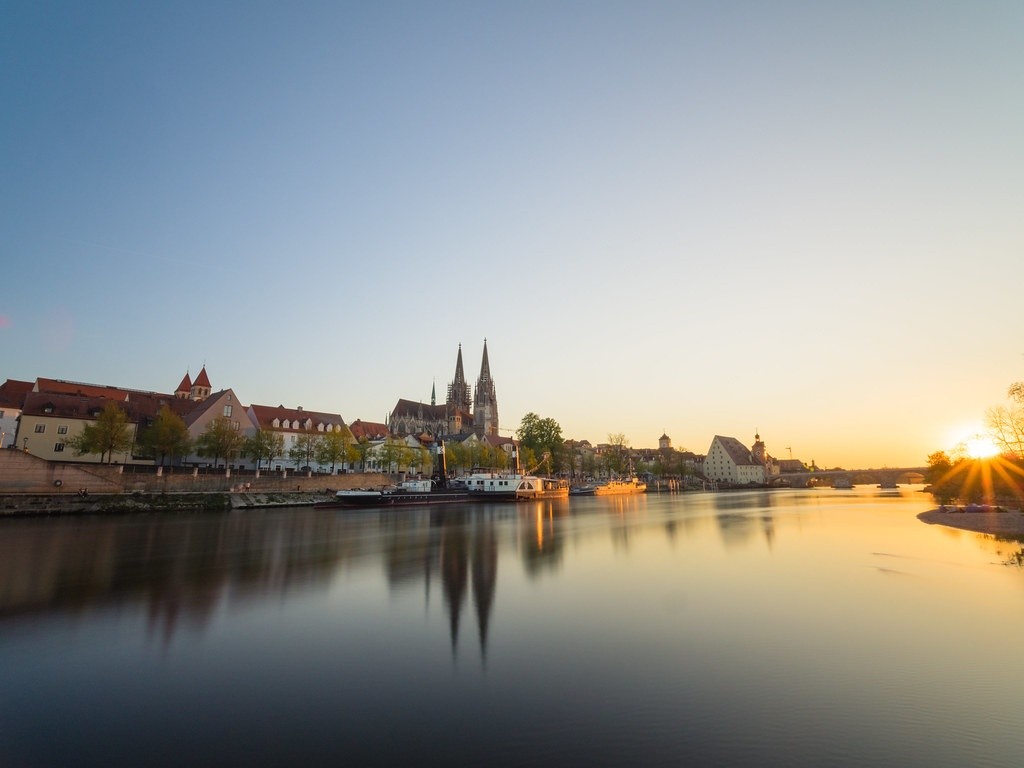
[594,458,647,496]
[876,481,899,488]
[831,477,855,488]
[515,452,569,499]
[336,473,432,504]
